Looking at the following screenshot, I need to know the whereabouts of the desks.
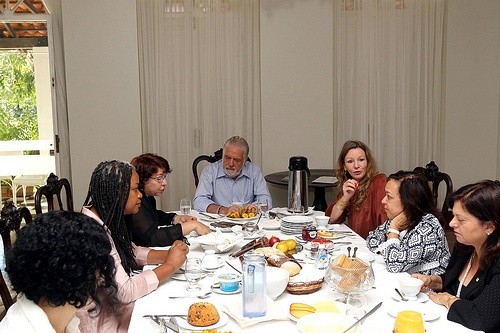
[264,168,339,212]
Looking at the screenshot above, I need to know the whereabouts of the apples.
[270,235,279,246]
[290,300,316,318]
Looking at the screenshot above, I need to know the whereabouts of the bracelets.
[335,199,349,211]
[445,295,457,309]
[216,206,225,215]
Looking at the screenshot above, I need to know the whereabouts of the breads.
[187,302,219,326]
[244,246,324,295]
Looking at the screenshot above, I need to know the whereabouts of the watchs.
[385,227,401,236]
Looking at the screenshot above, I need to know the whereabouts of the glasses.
[149,175,167,182]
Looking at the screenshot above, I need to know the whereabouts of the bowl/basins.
[296,311,362,333]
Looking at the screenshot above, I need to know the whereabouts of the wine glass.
[185,258,202,292]
[257,198,268,219]
[325,254,374,307]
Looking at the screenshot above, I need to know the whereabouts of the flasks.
[288,156,311,213]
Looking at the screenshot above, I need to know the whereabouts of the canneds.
[302,226,316,240]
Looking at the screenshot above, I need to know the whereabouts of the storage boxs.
[323,255,376,304]
[286,269,324,294]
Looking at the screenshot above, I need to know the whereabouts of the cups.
[180,199,191,216]
[346,291,367,325]
[218,273,240,291]
[399,278,424,300]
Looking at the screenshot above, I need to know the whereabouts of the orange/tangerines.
[277,239,297,252]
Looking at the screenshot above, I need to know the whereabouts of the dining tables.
[126,210,484,333]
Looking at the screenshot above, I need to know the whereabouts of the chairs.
[0,201,33,311]
[35,172,73,214]
[191,147,252,189]
[413,160,453,225]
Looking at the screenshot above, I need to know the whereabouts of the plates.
[387,301,441,322]
[288,296,358,321]
[173,309,228,330]
[170,265,207,281]
[178,205,352,271]
[392,291,429,303]
[211,282,242,295]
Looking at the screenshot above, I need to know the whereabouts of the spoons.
[368,257,375,276]
[395,287,408,301]
[169,289,212,298]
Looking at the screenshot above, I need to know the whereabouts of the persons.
[128,154,212,247]
[364,170,451,277]
[324,140,390,241]
[192,136,272,218]
[74,160,189,332]
[0,210,118,332]
[411,180,499,333]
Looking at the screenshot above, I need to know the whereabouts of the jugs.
[242,253,267,318]
